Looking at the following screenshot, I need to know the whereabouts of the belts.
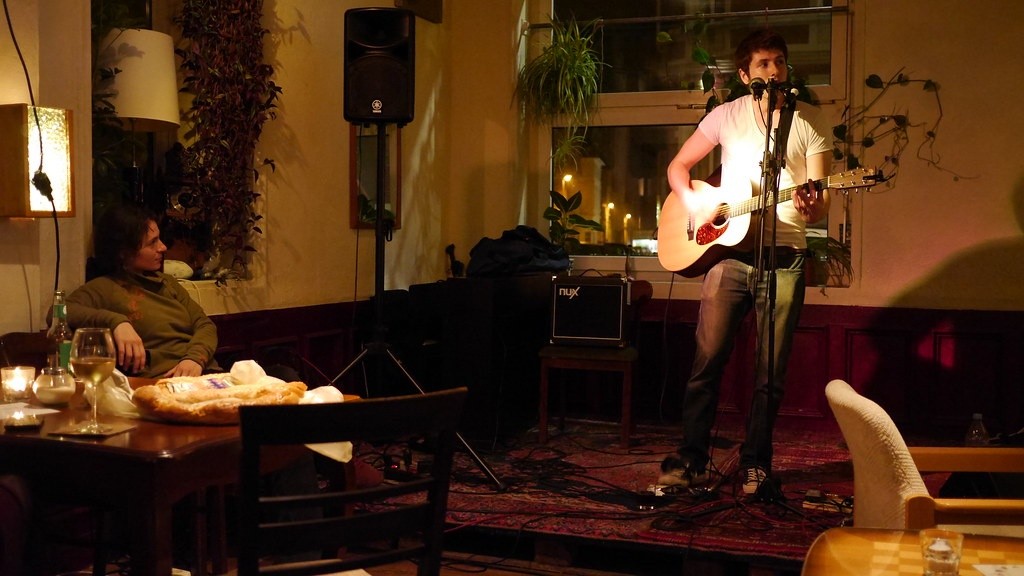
[749,246,809,256]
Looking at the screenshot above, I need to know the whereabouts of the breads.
[132,381,308,423]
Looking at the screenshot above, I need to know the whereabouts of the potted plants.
[511,8,615,174]
[805,228,855,297]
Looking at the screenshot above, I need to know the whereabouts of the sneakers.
[742,465,767,493]
[658,462,710,486]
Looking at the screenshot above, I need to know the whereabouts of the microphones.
[779,88,799,114]
[748,77,765,101]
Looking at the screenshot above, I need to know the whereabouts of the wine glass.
[70,328,116,433]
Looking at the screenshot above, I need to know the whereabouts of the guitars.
[656,164,878,279]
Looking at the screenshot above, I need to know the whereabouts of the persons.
[646,30,829,495]
[46,205,223,377]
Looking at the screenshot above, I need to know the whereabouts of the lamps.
[96,27,181,213]
[0,102,77,218]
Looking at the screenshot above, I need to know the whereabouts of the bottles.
[964,413,990,446]
[46,289,72,367]
[32,367,76,404]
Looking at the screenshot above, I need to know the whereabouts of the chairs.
[822,378,1024,538]
[0,331,470,576]
[537,279,655,454]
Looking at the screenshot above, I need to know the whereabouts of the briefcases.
[550,269,630,349]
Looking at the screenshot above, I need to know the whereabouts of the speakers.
[344,7,415,129]
[550,276,630,348]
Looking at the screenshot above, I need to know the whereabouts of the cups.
[2,366,36,403]
[919,528,963,576]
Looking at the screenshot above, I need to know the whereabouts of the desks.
[0,374,308,576]
[801,527,1024,576]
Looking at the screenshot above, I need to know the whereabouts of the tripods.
[687,77,816,523]
[329,124,506,493]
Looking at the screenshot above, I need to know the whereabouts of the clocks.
[165,142,207,230]
